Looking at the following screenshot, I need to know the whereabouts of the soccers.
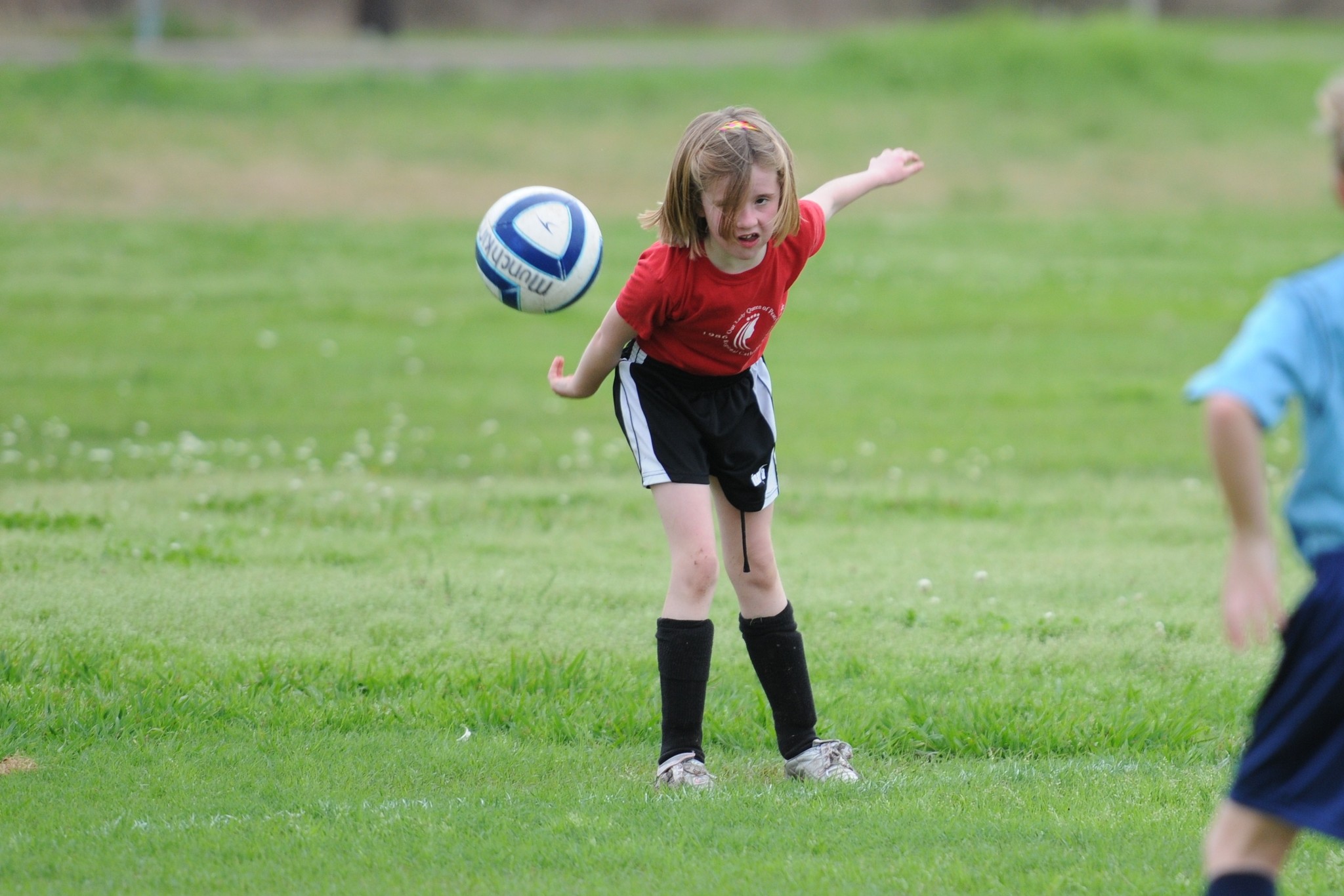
[472,186,605,317]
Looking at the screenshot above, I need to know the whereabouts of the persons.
[547,108,925,786]
[1180,72,1344,896]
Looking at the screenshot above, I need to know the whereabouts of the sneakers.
[652,754,715,795]
[784,736,860,784]
